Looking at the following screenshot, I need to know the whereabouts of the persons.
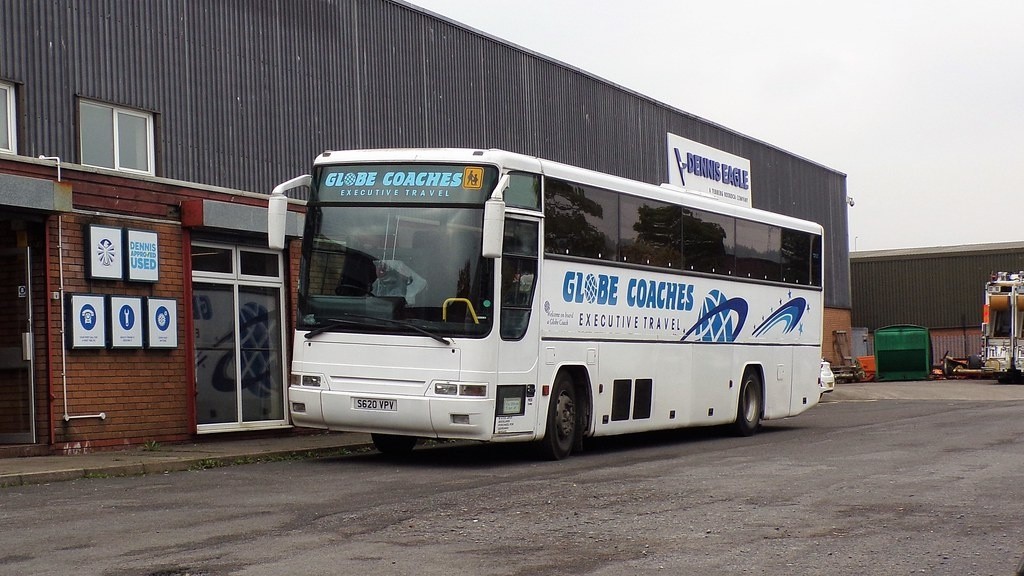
[627,236,667,266]
[669,245,695,271]
[427,264,487,323]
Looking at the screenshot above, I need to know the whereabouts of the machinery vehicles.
[981,269,1024,383]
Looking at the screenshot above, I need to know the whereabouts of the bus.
[268,149,825,455]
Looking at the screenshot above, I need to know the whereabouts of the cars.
[819,359,834,398]
